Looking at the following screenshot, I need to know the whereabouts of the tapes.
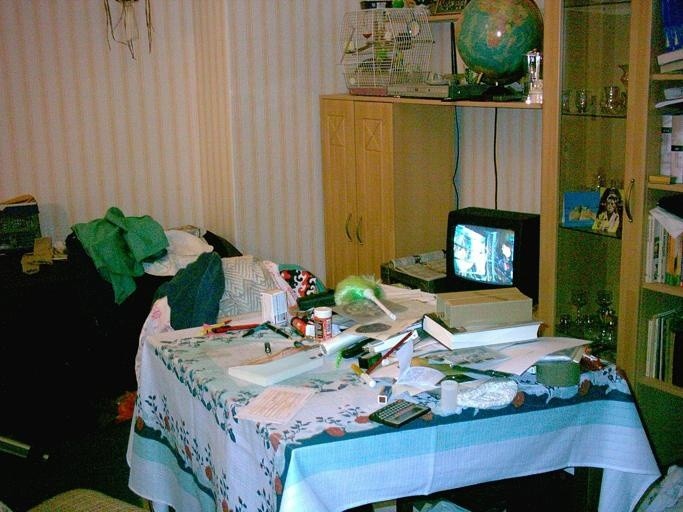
[536,364,581,387]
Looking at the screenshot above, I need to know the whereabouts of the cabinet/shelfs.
[535,0,683,466]
[319,93,455,289]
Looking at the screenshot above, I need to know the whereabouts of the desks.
[145,284,617,512]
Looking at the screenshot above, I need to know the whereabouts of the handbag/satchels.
[164,252,226,331]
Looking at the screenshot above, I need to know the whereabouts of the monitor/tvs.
[446,207,540,303]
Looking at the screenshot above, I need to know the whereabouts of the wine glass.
[571,290,589,327]
[595,289,617,344]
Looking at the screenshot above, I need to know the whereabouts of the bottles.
[313,306,331,343]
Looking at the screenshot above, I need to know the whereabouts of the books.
[227,344,324,387]
[0,193,42,254]
[422,313,545,351]
[644,0,682,390]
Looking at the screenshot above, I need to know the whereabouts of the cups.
[440,380,459,411]
[561,84,621,115]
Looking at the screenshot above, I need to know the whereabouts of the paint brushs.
[359,329,413,385]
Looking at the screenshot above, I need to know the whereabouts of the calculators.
[369,397,431,427]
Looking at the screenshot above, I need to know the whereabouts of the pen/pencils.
[206,319,232,333]
[351,363,378,389]
[266,322,290,338]
[212,323,262,334]
[242,320,270,337]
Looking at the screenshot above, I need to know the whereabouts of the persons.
[494,241,513,285]
[591,196,621,233]
